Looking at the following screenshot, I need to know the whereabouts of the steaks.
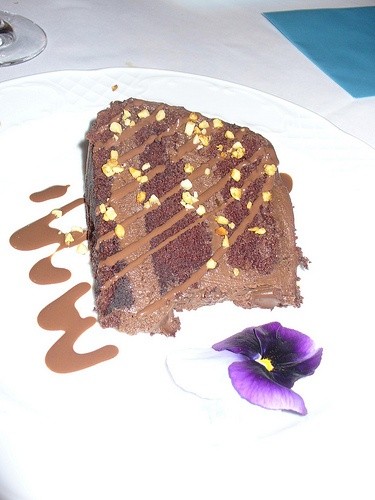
[84,97,310,337]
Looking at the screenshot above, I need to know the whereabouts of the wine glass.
[0,11,47,67]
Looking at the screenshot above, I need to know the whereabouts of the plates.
[1,68,375,497]
